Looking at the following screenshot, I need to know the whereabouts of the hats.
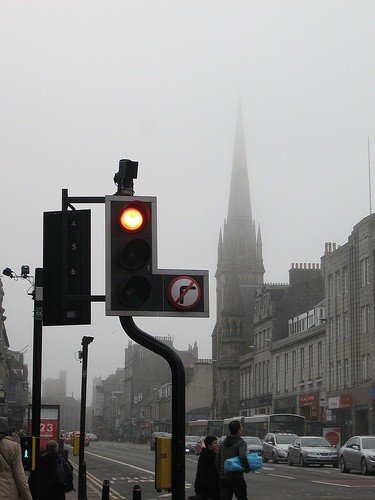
[46,440,58,454]
[0,417,9,433]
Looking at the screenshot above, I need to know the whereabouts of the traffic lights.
[105,196,208,317]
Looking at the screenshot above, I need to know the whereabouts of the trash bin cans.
[59,438,64,450]
[59,450,69,460]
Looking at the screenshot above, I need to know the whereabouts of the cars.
[65,431,98,446]
[288,436,338,467]
[151,432,262,456]
[339,435,375,474]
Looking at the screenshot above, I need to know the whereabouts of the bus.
[188,419,224,436]
[223,414,306,442]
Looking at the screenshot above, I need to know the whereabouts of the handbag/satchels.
[56,456,74,492]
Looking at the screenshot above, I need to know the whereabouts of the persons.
[0,416,32,500]
[215,421,250,500]
[11,427,25,442]
[194,436,218,500]
[28,440,74,500]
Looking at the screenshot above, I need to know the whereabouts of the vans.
[262,433,298,464]
